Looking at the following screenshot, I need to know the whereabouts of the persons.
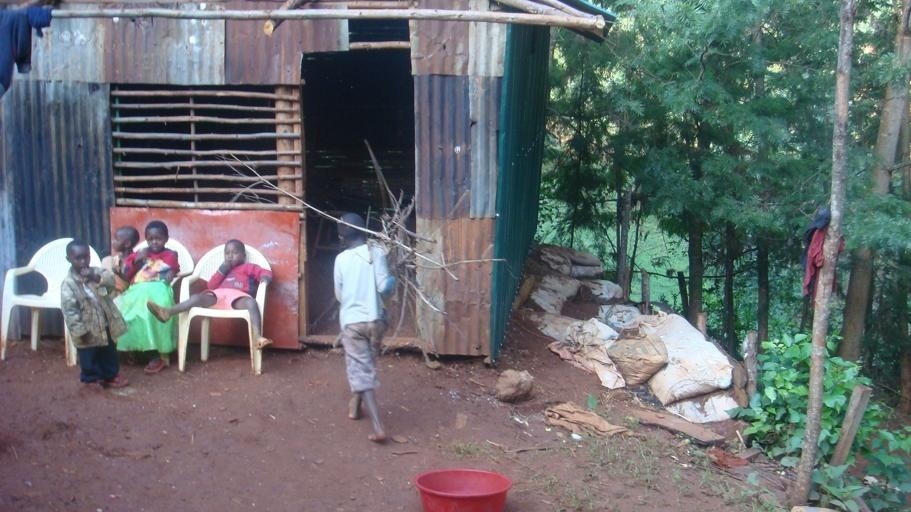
[147,239,274,350]
[111,221,181,373]
[59,238,129,391]
[107,224,140,296]
[333,212,395,441]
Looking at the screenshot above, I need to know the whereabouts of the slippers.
[146,301,168,324]
[144,358,165,374]
[255,336,274,350]
[103,375,130,388]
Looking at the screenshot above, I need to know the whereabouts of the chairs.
[115,238,194,367]
[178,244,272,374]
[0,238,100,367]
